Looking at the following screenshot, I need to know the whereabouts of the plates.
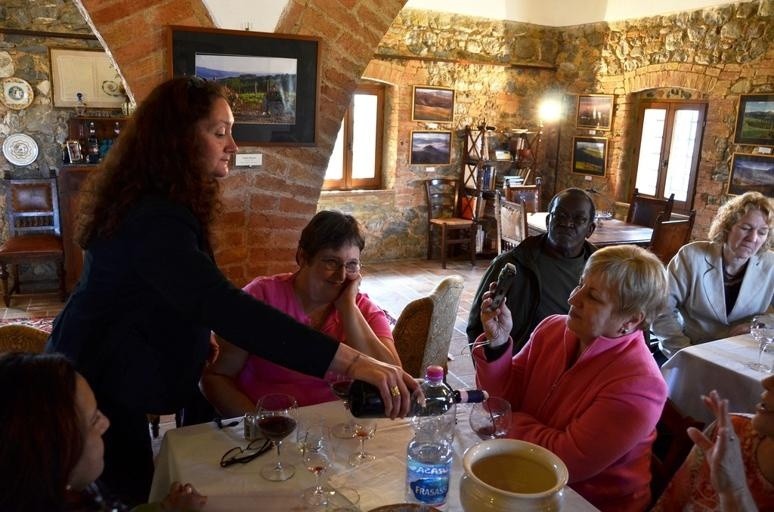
[367,503,441,512]
[1,132,39,167]
[1,76,35,110]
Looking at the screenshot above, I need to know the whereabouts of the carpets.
[0,316,55,333]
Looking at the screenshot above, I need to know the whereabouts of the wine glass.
[746,313,774,373]
[253,392,301,482]
[327,371,359,441]
[296,414,343,509]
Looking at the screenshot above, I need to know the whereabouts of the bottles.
[595,217,603,232]
[74,92,88,116]
[87,121,100,163]
[113,122,121,140]
[404,364,458,512]
[345,377,490,420]
[100,126,114,158]
[62,142,69,164]
[77,123,89,161]
[121,94,133,115]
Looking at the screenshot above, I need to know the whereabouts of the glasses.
[221,437,272,467]
[312,255,362,272]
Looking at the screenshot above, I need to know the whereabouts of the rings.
[729,435,735,441]
[390,384,400,397]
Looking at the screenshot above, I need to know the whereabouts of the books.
[460,131,531,253]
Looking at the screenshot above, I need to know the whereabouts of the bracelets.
[344,353,361,377]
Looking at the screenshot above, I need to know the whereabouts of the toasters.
[347,414,377,469]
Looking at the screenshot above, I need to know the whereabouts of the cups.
[468,396,514,439]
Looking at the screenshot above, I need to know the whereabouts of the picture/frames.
[409,130,453,167]
[570,136,609,178]
[726,152,774,197]
[732,92,774,147]
[163,22,322,147]
[575,94,615,131]
[410,85,457,124]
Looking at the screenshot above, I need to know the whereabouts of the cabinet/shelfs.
[455,118,544,257]
[60,116,129,271]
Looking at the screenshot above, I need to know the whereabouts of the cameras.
[243,412,280,442]
[490,262,517,312]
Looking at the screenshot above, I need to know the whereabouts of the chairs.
[393,275,465,381]
[0,169,67,307]
[423,178,696,268]
[0,325,50,357]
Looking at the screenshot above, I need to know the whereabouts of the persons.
[197,210,403,419]
[472,244,670,512]
[0,351,209,512]
[41,76,426,509]
[650,374,774,512]
[650,192,774,370]
[466,188,649,368]
